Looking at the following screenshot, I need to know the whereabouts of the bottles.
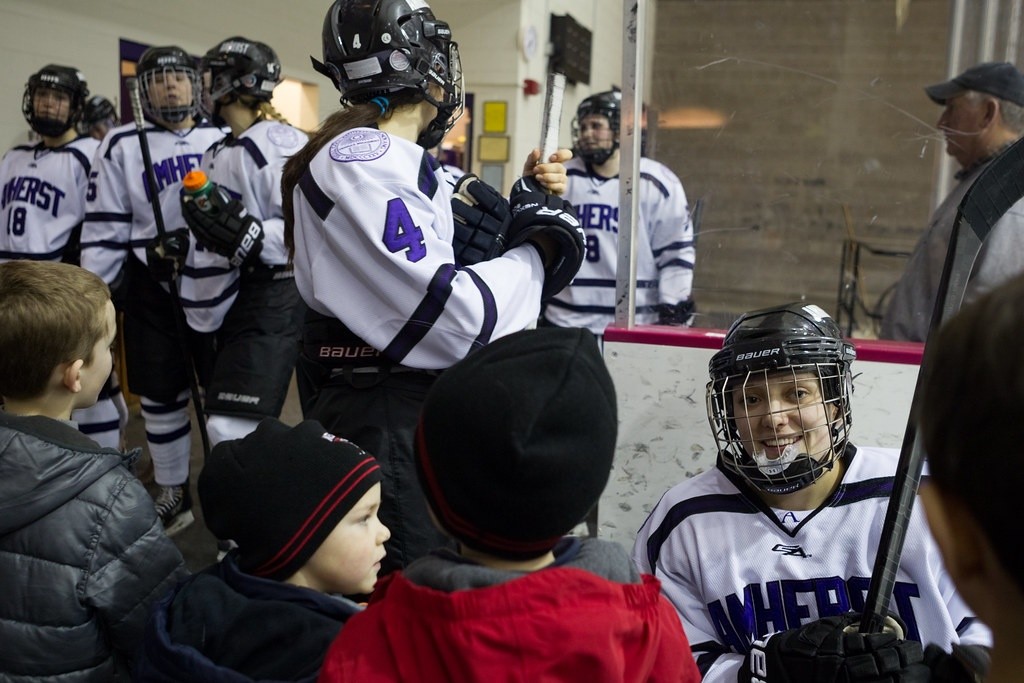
[184,170,222,221]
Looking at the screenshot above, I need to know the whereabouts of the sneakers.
[154,482,196,537]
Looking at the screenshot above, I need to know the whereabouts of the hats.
[412,328,618,562]
[924,61,1024,107]
[199,417,383,581]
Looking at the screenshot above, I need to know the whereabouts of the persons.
[82,96,118,141]
[877,62,1024,344]
[179,36,309,456]
[917,274,1024,683]
[539,87,696,331]
[0,261,192,683]
[115,416,390,683]
[630,301,991,683]
[282,0,586,574]
[0,64,142,472]
[78,46,231,538]
[318,328,700,683]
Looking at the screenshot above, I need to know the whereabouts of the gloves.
[451,174,512,266]
[507,175,587,301]
[742,611,931,683]
[179,188,264,271]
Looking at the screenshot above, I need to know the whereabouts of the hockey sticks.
[861,133,1024,630]
[536,70,569,176]
[124,76,210,458]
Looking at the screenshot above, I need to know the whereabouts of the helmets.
[572,91,622,164]
[83,98,118,140]
[22,64,91,136]
[309,0,465,150]
[707,302,857,495]
[196,36,286,127]
[137,46,198,122]
